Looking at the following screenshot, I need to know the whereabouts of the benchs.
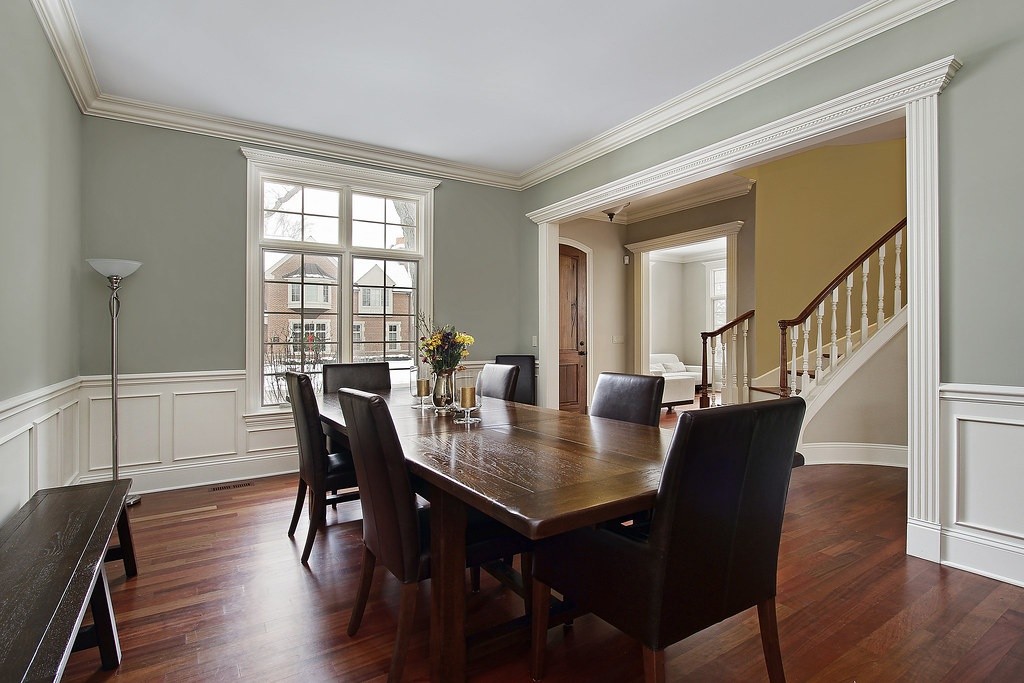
[0,478,139,683]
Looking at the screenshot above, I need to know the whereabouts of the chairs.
[590,372,665,428]
[475,364,520,401]
[495,355,535,405]
[285,370,358,562]
[322,362,392,455]
[533,396,806,683]
[337,387,532,683]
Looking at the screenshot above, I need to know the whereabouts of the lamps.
[85,258,143,507]
[601,201,631,222]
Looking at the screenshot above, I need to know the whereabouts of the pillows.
[650,363,667,374]
[663,362,686,372]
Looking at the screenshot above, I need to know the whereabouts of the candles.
[461,387,476,408]
[417,379,430,396]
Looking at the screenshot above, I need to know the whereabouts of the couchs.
[650,353,713,414]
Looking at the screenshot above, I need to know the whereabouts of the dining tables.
[285,384,806,683]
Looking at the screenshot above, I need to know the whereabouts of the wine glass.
[453,372,482,423]
[410,366,434,408]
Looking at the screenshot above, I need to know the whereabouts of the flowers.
[414,312,475,372]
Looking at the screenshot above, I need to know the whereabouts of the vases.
[446,371,456,405]
[433,374,447,407]
